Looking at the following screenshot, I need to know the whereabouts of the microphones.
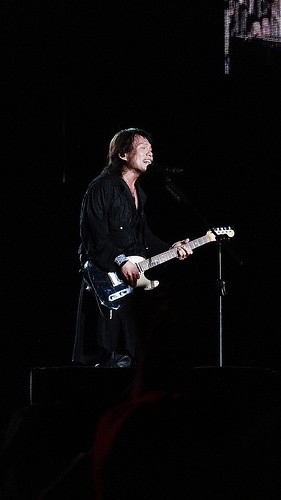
[152,163,176,173]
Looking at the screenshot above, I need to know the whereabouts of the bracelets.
[115,255,128,266]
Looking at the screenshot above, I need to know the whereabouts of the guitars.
[84,225,236,311]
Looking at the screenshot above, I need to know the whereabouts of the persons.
[72,128,192,365]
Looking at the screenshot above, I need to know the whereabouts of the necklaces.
[130,187,136,197]
